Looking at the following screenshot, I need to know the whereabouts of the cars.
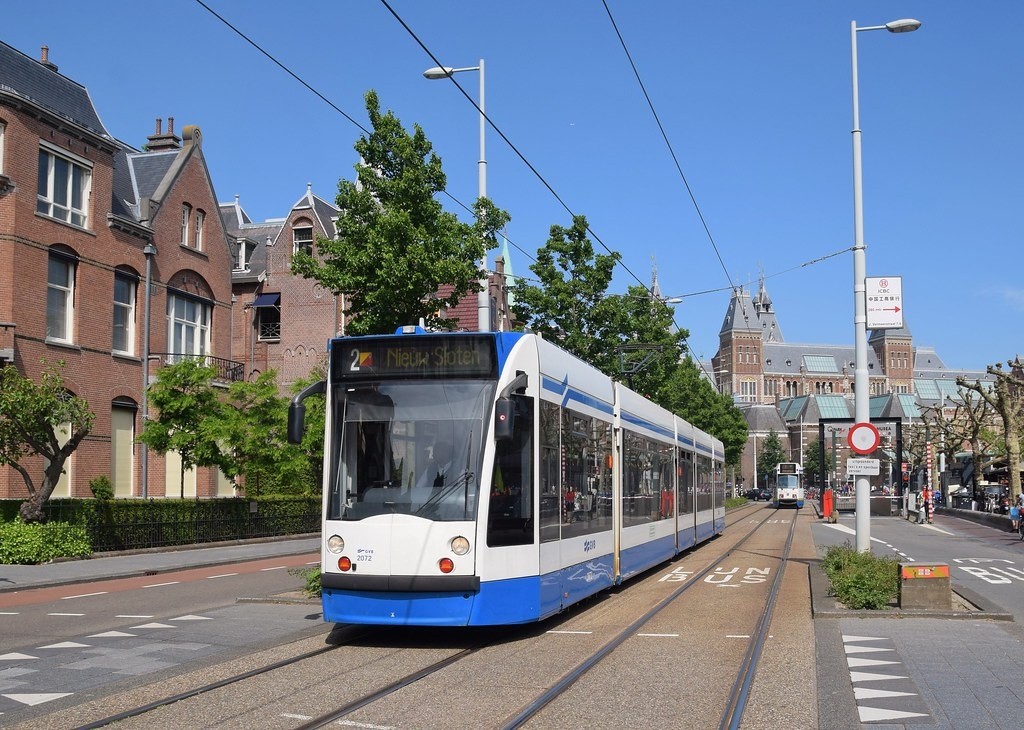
[744,488,772,501]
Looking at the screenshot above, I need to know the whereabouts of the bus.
[287,322,726,627]
[910,450,1008,512]
[726,483,732,496]
[772,464,805,509]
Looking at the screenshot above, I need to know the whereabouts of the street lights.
[424,65,492,332]
[651,298,683,305]
[850,18,924,555]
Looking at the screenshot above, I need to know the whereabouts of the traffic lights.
[903,473,908,487]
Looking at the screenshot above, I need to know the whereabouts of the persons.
[871,483,877,491]
[843,482,849,493]
[917,485,932,508]
[662,486,673,518]
[735,484,740,498]
[587,490,595,521]
[985,494,1024,530]
[804,487,820,500]
[882,483,894,496]
[416,441,465,488]
[567,486,584,523]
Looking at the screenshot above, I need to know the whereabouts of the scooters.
[984,488,1009,515]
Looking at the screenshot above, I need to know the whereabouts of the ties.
[442,470,444,475]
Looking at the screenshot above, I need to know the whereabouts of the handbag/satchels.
[1009,505,1020,521]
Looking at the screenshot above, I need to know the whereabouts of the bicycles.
[1014,505,1024,540]
